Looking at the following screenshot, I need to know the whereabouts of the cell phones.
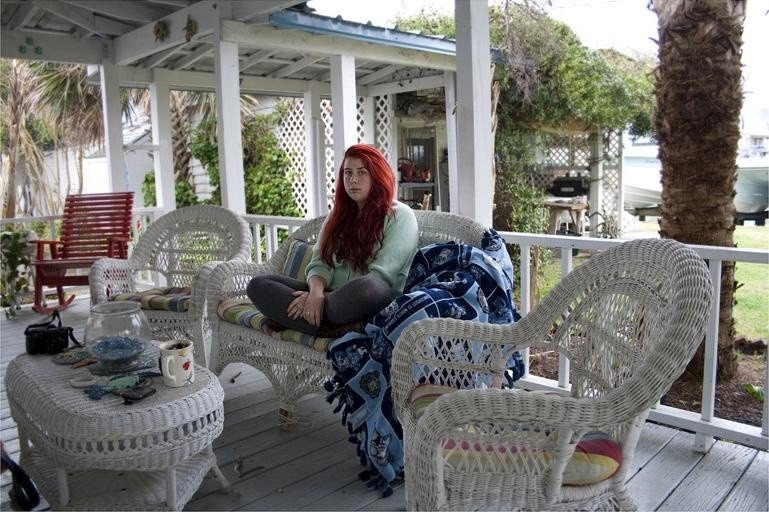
[119,388,156,400]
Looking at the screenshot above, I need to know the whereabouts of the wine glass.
[85,301,153,371]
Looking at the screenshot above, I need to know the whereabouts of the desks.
[4,337,241,512]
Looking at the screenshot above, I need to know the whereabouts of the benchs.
[204,209,515,433]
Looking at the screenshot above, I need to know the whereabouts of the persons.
[241,143,419,339]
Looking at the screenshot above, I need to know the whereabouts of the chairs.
[88,204,253,368]
[26,190,135,315]
[388,236,715,511]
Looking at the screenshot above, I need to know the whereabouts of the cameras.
[26,327,68,355]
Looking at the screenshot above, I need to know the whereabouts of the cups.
[158,339,195,387]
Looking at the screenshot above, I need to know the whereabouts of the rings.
[305,311,310,316]
